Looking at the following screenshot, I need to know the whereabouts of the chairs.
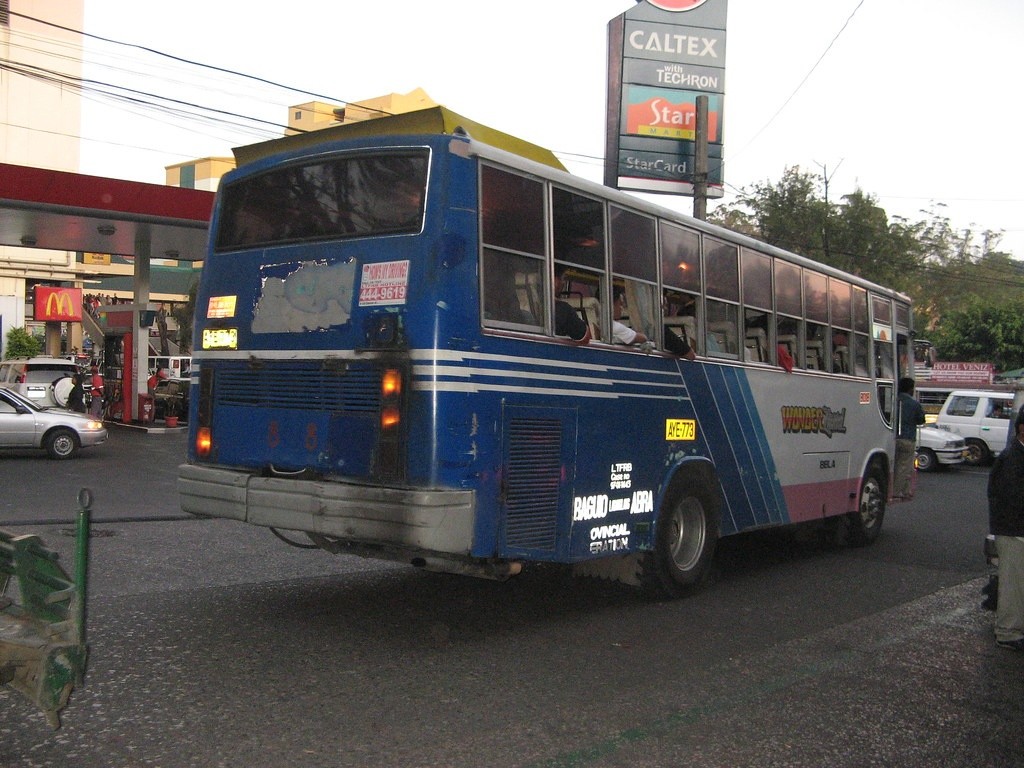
[480,272,867,379]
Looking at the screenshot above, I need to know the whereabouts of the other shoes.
[893,495,913,501]
[996,640,1024,654]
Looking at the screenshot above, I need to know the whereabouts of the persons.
[371,177,425,234]
[834,334,867,377]
[988,403,1024,646]
[83,293,121,319]
[182,362,190,376]
[988,405,1003,419]
[90,366,104,421]
[148,367,167,394]
[66,374,86,413]
[894,378,926,498]
[484,242,796,374]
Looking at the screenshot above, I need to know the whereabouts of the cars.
[0,385,109,462]
[914,425,970,473]
[58,352,191,426]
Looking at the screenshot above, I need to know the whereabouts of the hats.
[90,366,98,371]
[157,370,166,379]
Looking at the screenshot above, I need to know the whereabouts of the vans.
[935,390,1015,468]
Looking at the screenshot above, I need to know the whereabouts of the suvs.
[0,357,91,412]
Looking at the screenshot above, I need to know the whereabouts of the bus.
[173,106,940,601]
